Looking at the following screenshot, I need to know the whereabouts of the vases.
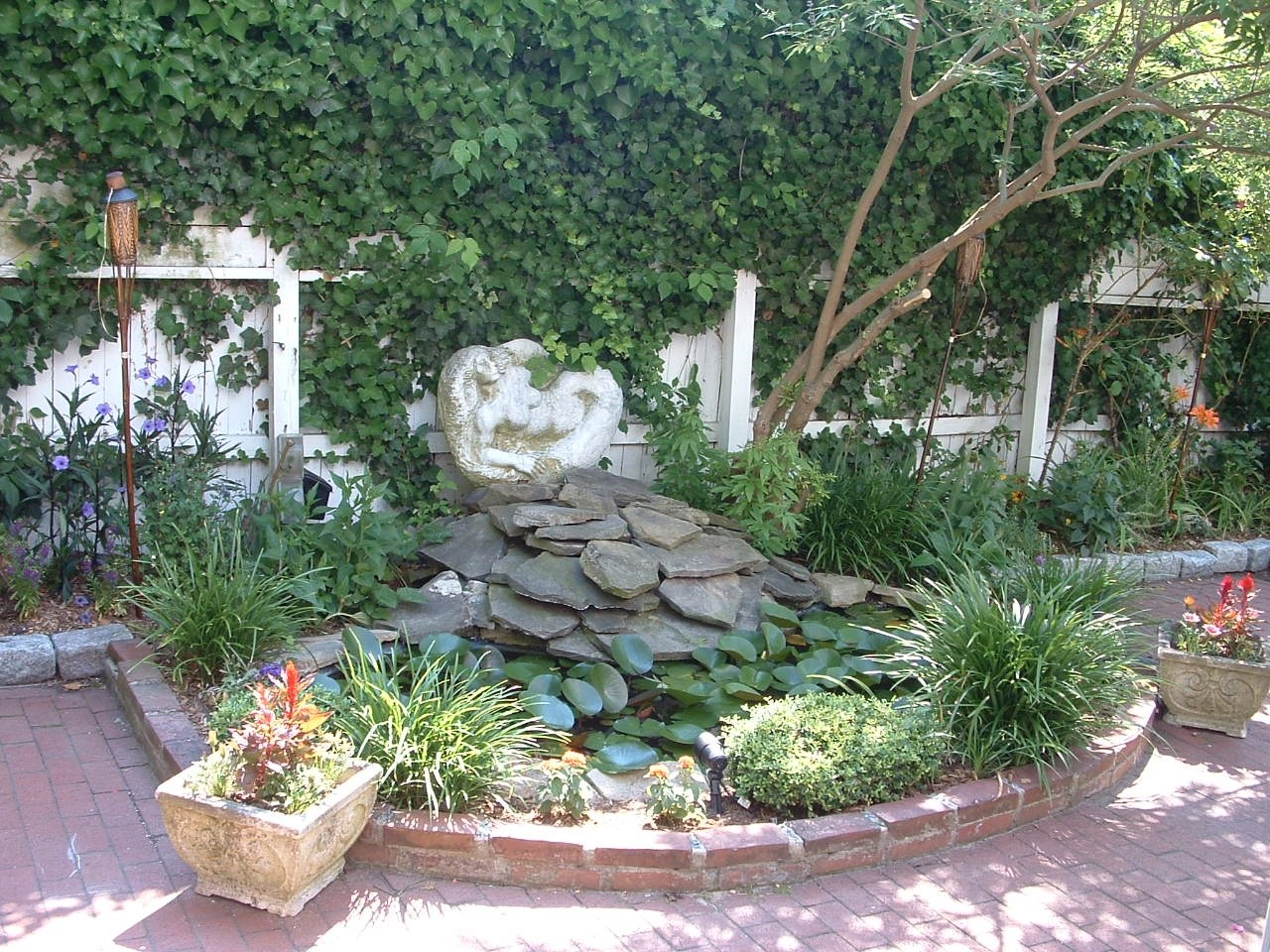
[1157,627,1270,738]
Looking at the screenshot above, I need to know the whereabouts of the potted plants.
[154,658,382,917]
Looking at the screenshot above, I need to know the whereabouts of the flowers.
[1173,572,1270,665]
